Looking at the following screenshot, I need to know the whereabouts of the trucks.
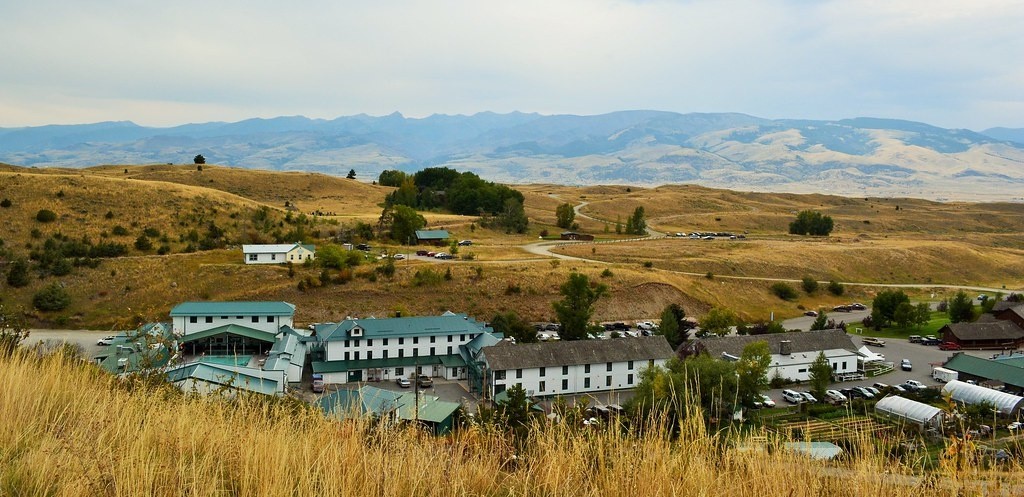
[930,367,958,383]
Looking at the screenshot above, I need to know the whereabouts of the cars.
[742,375,929,410]
[665,230,745,241]
[977,293,988,300]
[1007,421,1024,432]
[395,377,411,388]
[803,310,818,317]
[533,320,660,343]
[310,379,326,393]
[354,244,458,260]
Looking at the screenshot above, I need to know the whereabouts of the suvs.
[862,336,886,348]
[900,358,913,371]
[910,334,943,346]
[458,240,473,246]
[97,335,116,346]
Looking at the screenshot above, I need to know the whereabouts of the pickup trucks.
[847,303,867,311]
[417,373,434,388]
[937,341,962,351]
[833,305,853,312]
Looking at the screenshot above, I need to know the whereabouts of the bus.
[581,405,610,420]
[605,404,625,417]
